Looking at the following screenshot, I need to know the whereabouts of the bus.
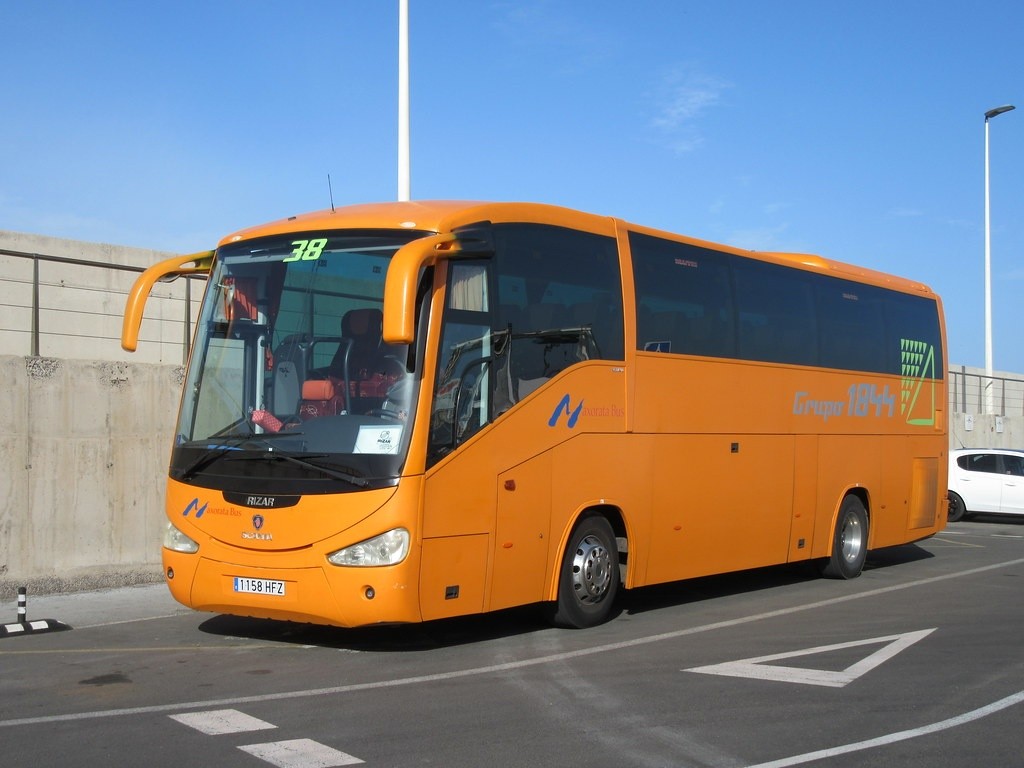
[122,199,950,629]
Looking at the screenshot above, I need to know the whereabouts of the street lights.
[984,105,1018,412]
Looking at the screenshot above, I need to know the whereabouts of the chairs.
[327,308,383,380]
[503,302,689,369]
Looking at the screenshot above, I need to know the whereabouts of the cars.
[948,448,1024,523]
